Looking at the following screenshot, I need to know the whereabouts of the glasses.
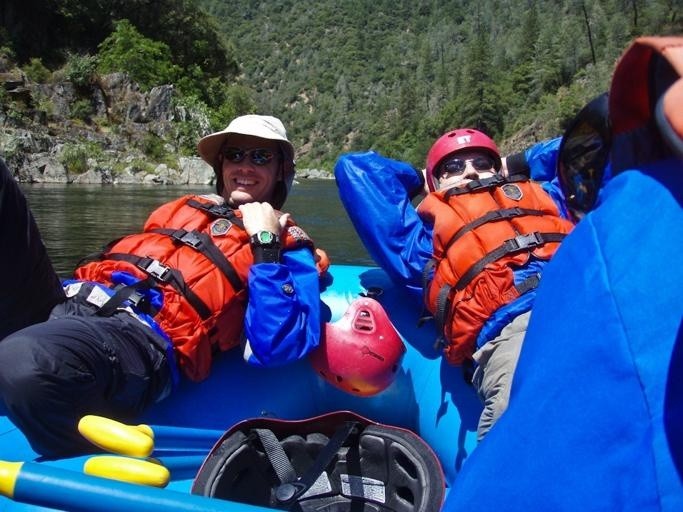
[439,157,496,176]
[220,145,274,170]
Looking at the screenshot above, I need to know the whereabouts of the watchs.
[250,231,282,248]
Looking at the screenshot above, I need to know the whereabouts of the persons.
[336,32,683,512]
[0,114,322,459]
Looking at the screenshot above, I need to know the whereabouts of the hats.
[196,113,294,173]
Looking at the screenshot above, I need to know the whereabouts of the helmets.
[192,410,447,512]
[425,127,499,196]
[311,296,407,398]
